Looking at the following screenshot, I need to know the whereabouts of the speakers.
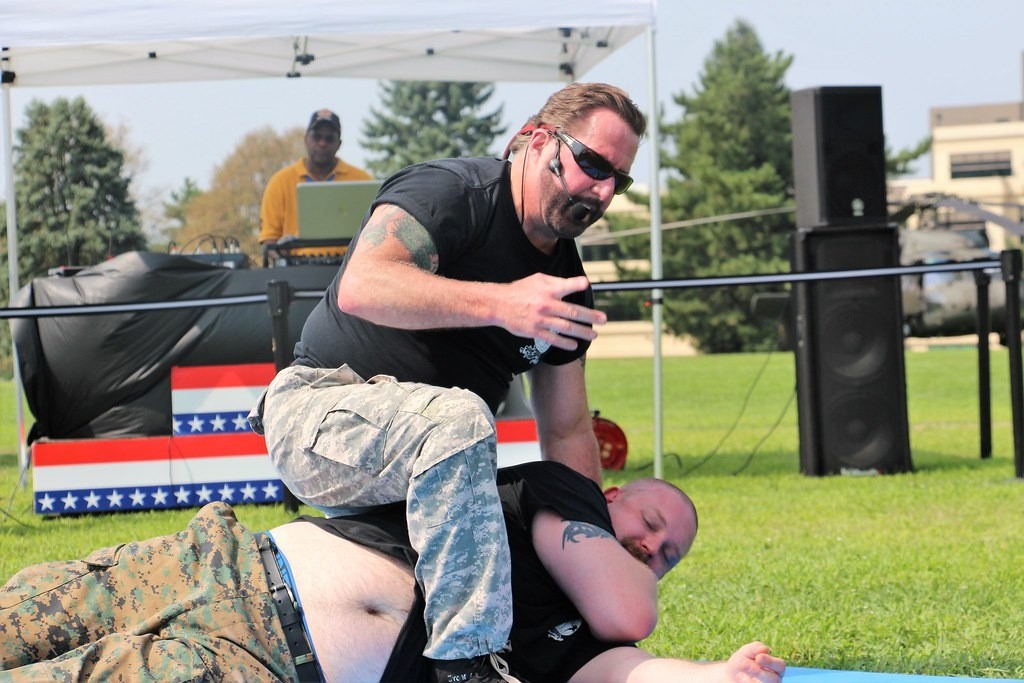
[787,228,913,476]
[791,85,888,228]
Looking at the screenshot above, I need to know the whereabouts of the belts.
[253,531,322,683]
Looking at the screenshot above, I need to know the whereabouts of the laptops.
[296,180,384,238]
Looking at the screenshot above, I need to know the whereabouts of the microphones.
[549,159,588,220]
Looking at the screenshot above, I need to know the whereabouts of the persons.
[248,84,645,683]
[0,460,788,683]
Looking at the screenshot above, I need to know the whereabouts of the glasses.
[309,131,339,144]
[555,129,634,196]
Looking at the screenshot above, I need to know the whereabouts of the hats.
[308,109,342,134]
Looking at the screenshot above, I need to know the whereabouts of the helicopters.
[579,193,1024,341]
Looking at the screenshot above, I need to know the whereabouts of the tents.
[1,1,664,484]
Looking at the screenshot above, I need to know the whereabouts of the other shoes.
[432,657,536,683]
